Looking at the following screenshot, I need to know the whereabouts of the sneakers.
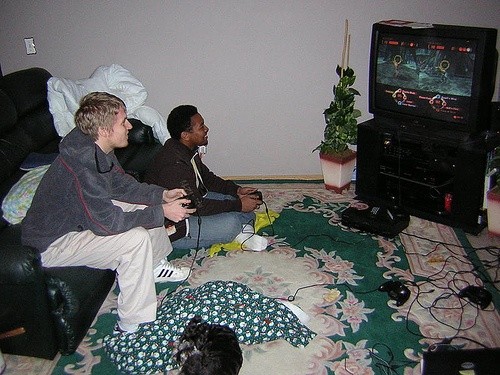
[114,323,128,335]
[153,257,193,283]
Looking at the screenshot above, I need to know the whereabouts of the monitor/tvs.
[368,20,498,142]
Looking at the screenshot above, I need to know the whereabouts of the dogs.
[173,314,244,375]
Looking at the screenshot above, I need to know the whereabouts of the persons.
[22,91,196,334]
[141,105,263,250]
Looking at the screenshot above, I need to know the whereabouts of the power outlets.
[24,38,37,55]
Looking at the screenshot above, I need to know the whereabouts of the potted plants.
[312,19,361,193]
[486,145,500,238]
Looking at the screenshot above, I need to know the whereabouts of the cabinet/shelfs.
[357,119,500,234]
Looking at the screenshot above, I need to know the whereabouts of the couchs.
[0,66,161,359]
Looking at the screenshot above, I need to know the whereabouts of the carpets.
[44,179,500,375]
